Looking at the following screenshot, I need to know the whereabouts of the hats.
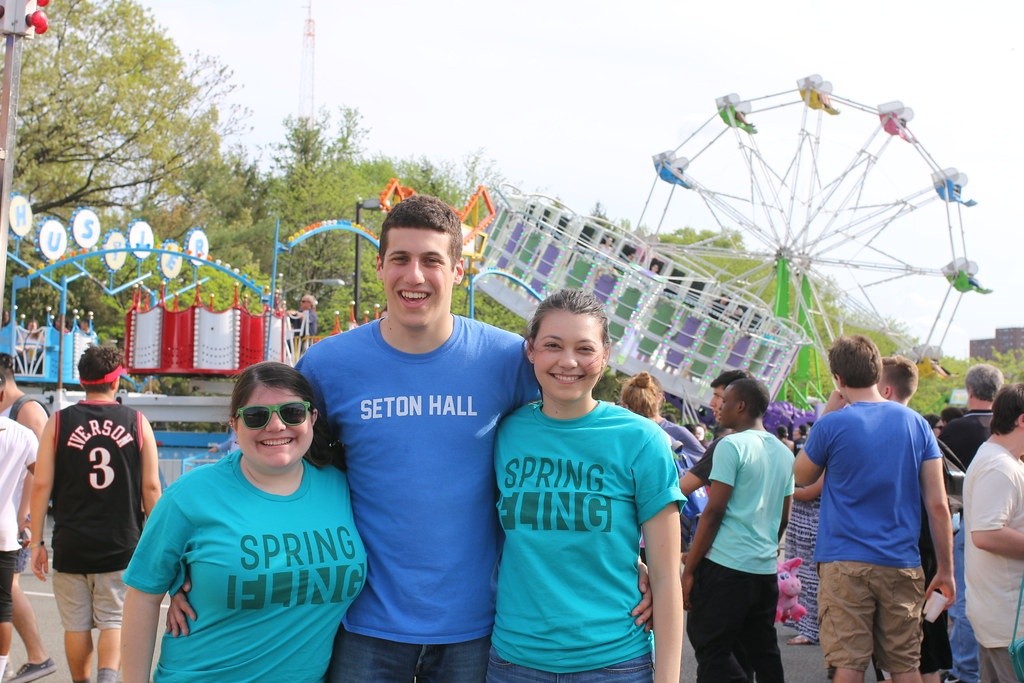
[79,364,135,386]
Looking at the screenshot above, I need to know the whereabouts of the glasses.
[235,401,316,430]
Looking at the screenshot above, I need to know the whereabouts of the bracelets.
[29,540,45,550]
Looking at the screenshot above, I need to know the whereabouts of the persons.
[620,335,1024,682]
[31,346,163,683]
[0,308,97,374]
[120,360,368,683]
[0,371,39,682]
[286,295,319,337]
[162,195,654,683]
[0,351,57,683]
[485,287,689,683]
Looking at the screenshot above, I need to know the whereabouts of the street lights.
[355,199,381,320]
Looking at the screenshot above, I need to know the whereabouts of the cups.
[923,590,949,623]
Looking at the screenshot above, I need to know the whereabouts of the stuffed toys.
[770,557,807,622]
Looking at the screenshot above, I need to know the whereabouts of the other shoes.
[787,635,814,645]
[941,674,966,683]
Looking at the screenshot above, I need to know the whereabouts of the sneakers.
[8,658,57,683]
